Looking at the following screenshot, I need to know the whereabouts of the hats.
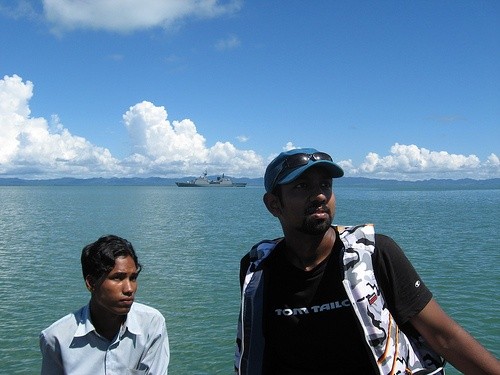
[264,148,344,192]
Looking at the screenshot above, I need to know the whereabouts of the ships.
[175,169,247,187]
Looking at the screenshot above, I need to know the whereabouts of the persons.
[233,148,500,375]
[38,234,171,375]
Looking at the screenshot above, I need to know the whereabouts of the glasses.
[271,152,333,196]
